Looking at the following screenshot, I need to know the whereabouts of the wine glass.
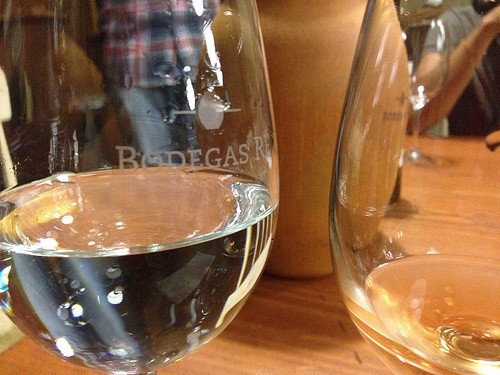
[401,19,450,167]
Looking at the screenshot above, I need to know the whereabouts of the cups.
[327,0,500,375]
[0,0,281,375]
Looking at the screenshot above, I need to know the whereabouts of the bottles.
[194,0,409,280]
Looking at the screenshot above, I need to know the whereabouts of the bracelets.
[462,38,478,73]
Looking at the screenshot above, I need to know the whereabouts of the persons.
[399,0,499,137]
[93,0,219,168]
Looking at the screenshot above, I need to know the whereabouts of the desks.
[2,132,500,375]
